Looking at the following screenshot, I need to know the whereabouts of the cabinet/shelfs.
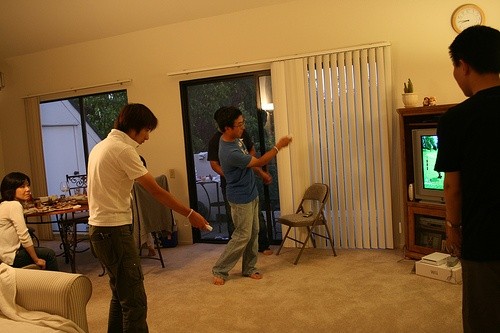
[394,103,459,260]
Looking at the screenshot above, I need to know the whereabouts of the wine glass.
[60,181,70,198]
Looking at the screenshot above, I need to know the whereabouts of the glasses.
[230,123,246,128]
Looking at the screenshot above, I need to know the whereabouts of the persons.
[434,25,500,333]
[87,103,213,333]
[0,172,58,273]
[210,106,292,285]
[207,127,272,256]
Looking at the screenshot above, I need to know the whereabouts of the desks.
[23,205,88,273]
[197,176,225,232]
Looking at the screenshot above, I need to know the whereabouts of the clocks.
[450,2,488,35]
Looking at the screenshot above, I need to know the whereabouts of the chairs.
[98,175,179,277]
[58,174,98,259]
[277,183,337,266]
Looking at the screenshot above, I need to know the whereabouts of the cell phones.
[303,211,313,217]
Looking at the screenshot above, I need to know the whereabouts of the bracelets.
[274,146,278,153]
[187,209,193,219]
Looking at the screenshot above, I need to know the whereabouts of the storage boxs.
[415,260,462,284]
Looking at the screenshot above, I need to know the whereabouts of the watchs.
[446,220,462,229]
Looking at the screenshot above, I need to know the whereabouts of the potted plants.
[400,77,419,106]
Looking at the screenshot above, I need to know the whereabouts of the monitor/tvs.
[411,128,445,202]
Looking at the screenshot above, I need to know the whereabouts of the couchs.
[0,267,93,333]
